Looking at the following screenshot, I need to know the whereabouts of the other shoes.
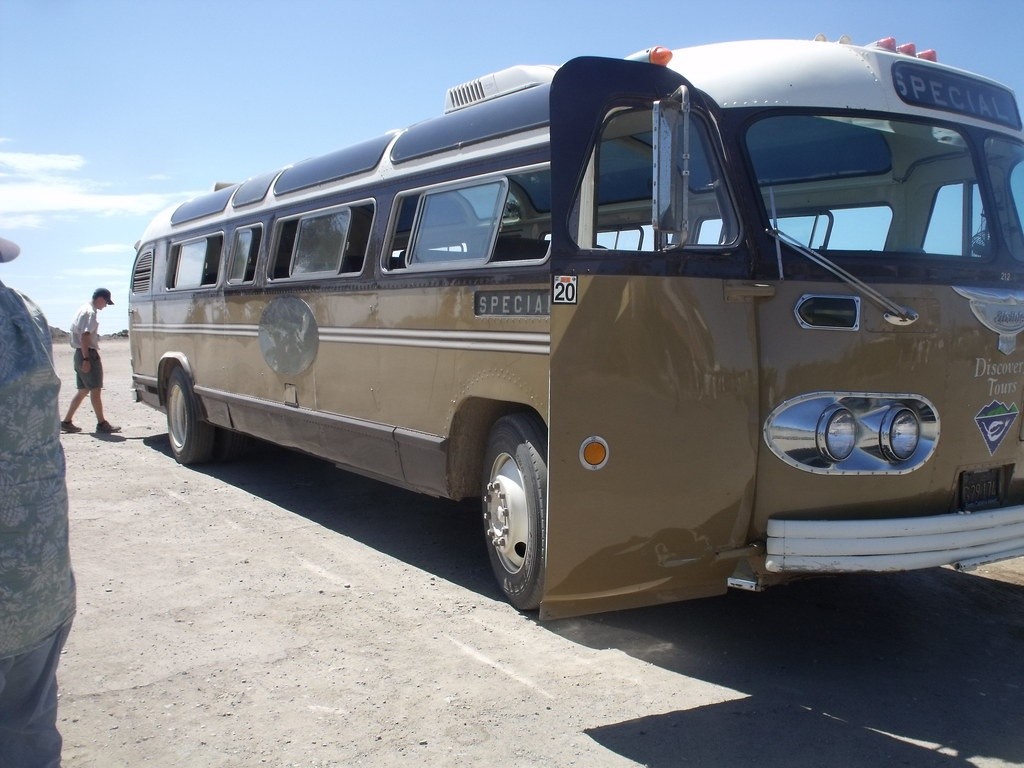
[97,421,121,432]
[60,420,82,432]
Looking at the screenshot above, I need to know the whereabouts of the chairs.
[336,256,397,274]
[482,235,550,262]
[396,250,479,270]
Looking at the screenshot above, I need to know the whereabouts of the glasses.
[104,297,109,304]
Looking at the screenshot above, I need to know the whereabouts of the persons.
[60,288,121,434]
[0,237,78,768]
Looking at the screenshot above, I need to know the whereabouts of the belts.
[77,348,94,352]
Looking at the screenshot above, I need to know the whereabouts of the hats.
[94,288,114,305]
[0,236,20,263]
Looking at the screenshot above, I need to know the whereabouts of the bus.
[127,35,1024,626]
